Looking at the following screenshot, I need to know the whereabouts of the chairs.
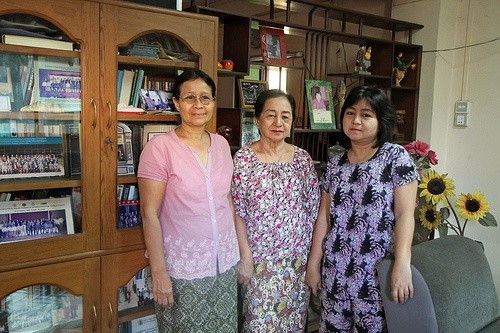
[377,235,500,333]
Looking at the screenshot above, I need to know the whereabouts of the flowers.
[404,142,497,250]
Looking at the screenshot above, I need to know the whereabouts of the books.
[134,33,179,50]
[117,70,174,107]
[2,34,74,51]
[0,55,34,112]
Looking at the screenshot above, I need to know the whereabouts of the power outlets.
[453,101,469,127]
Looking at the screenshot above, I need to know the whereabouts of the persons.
[268,40,280,54]
[354,44,371,72]
[305,85,421,333]
[393,52,408,87]
[42,78,81,93]
[62,290,78,319]
[312,91,326,111]
[119,286,153,303]
[1,216,64,239]
[118,147,124,161]
[0,154,61,174]
[137,69,240,333]
[8,309,47,331]
[231,89,321,333]
[119,211,140,227]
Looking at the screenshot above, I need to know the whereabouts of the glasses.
[179,95,214,104]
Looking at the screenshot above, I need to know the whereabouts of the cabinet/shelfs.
[196,0,425,163]
[0,0,217,333]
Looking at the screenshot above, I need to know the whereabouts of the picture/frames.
[305,80,336,129]
[0,119,69,178]
[33,64,83,104]
[238,79,269,109]
[260,26,288,66]
